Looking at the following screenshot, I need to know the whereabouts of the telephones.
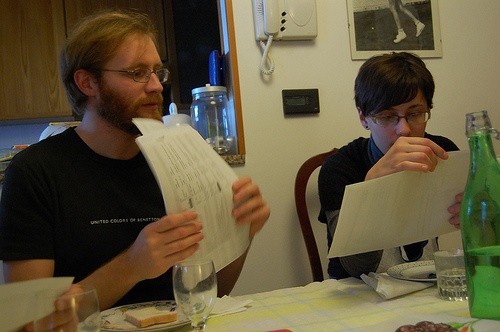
[252,0,318,42]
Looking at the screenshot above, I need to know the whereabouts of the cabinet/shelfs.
[0,0,179,121]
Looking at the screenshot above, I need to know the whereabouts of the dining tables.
[77,271,500,332]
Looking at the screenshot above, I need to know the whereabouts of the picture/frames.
[346,0,443,60]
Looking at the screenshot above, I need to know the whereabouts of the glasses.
[100,64,170,84]
[368,106,431,128]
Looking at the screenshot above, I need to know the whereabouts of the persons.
[317,52,465,280]
[0,8,270,332]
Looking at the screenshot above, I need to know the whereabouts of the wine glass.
[173,258,217,332]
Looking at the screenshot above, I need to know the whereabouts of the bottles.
[190,86,233,155]
[459,109,500,320]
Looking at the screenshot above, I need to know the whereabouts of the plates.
[347,313,500,332]
[387,260,437,282]
[84,300,192,332]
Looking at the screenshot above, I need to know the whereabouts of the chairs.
[294,150,334,282]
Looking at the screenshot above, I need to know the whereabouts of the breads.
[125,306,177,328]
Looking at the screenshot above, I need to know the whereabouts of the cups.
[32,286,100,332]
[432,248,469,301]
